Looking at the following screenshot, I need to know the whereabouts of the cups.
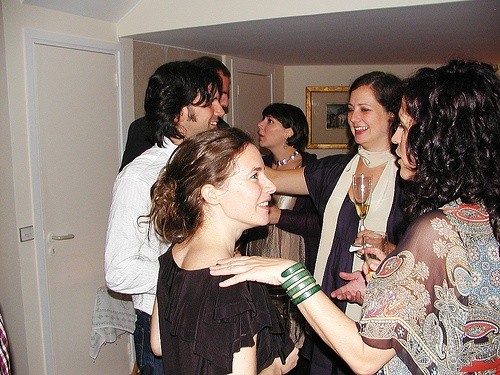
[362,231,389,280]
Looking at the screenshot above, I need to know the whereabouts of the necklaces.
[272,151,299,167]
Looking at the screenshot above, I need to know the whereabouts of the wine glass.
[352,173,373,247]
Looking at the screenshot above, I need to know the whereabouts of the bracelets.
[281,262,321,306]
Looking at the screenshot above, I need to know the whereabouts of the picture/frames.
[305,85,357,149]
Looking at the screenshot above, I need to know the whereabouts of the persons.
[210,60,500,375]
[104,56,407,375]
